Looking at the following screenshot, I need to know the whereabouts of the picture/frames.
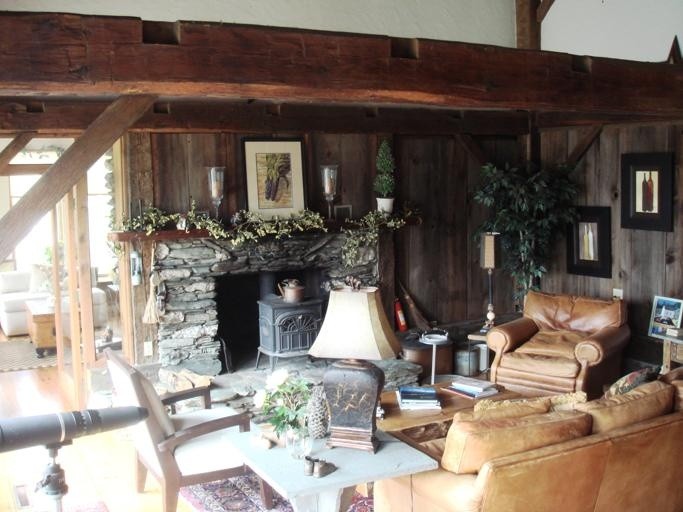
[565,206,610,278]
[620,152,675,232]
[235,137,310,222]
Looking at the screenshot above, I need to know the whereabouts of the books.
[439,375,502,402]
[394,381,443,411]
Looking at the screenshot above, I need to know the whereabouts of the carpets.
[177,472,373,512]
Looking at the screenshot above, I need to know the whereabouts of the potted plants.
[372,140,396,218]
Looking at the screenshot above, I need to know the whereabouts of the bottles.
[394,298,407,333]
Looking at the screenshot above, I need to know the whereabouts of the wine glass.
[318,164,340,219]
[203,164,229,219]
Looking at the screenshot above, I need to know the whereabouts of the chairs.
[102,349,275,512]
[0,267,108,359]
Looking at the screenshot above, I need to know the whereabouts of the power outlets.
[612,288,623,300]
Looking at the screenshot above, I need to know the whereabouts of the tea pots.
[276,278,306,303]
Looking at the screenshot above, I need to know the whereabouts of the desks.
[228,427,438,511]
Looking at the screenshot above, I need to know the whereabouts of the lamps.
[478,231,501,333]
[307,285,402,455]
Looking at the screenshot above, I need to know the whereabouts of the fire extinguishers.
[393,295,408,333]
